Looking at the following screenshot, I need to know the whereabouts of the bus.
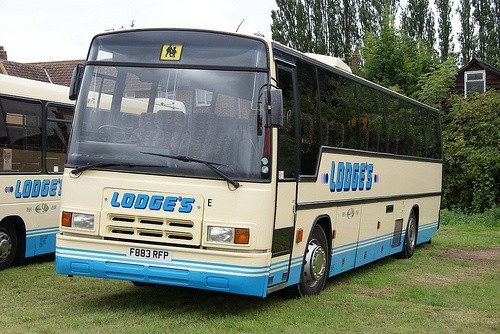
[0,71,187,270]
[55,27,444,299]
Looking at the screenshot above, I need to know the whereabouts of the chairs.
[148,108,440,179]
